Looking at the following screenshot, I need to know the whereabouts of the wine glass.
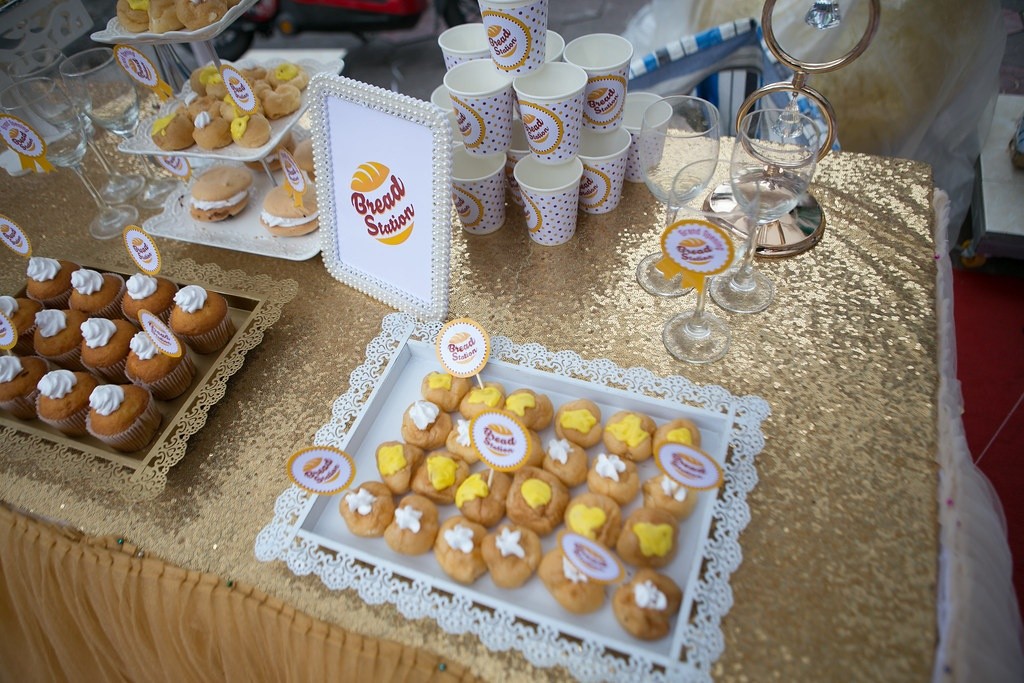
[638,95,721,298]
[0,77,139,240]
[709,108,822,315]
[6,48,147,204]
[663,159,760,365]
[60,48,184,209]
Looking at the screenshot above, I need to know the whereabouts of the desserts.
[0,256,236,452]
[187,128,320,239]
[339,370,702,641]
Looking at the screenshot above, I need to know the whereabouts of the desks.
[0,77,1024,683]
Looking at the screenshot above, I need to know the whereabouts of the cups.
[430,0,674,247]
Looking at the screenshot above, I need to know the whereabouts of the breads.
[150,62,307,151]
[116,0,242,34]
